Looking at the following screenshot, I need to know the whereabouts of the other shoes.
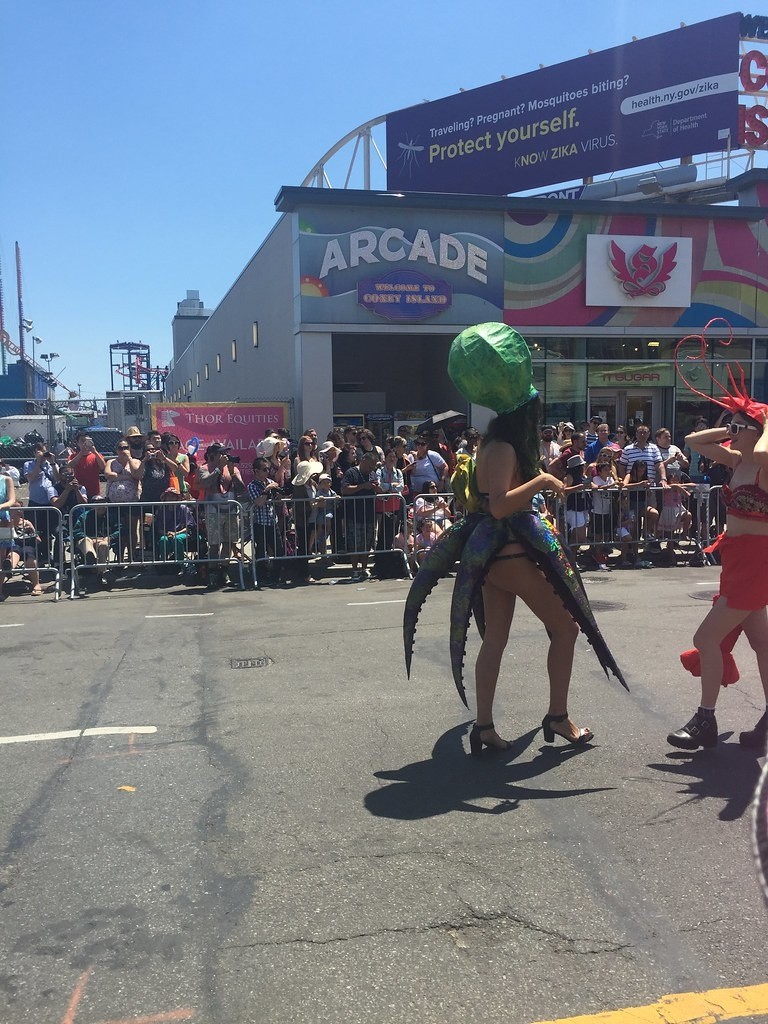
[304,576,314,583]
[86,550,95,576]
[98,575,110,590]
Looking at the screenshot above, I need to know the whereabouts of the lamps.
[178,388,181,399]
[182,384,186,396]
[204,364,209,380]
[196,372,200,387]
[216,353,221,373]
[168,392,177,403]
[231,339,236,363]
[638,175,663,195]
[252,321,259,348]
[188,379,192,392]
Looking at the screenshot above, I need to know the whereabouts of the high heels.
[470,723,515,757]
[541,714,595,745]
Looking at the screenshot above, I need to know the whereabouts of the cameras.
[43,451,51,457]
[149,453,156,458]
[226,454,241,463]
[271,486,284,496]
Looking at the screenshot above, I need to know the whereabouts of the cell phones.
[86,438,92,448]
[67,475,74,482]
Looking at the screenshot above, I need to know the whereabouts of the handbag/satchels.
[400,484,409,495]
[375,492,400,512]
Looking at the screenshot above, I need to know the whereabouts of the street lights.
[40,352,60,371]
[32,335,42,367]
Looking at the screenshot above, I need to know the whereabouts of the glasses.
[116,446,127,450]
[303,442,315,445]
[724,422,756,437]
[430,486,437,489]
[258,467,269,471]
[564,429,572,432]
[415,441,429,446]
[403,444,406,448]
[359,436,368,441]
[616,430,625,434]
[168,441,179,446]
[600,453,611,459]
[352,431,356,435]
[594,422,600,424]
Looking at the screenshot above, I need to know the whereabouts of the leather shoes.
[667,713,717,750]
[739,713,767,747]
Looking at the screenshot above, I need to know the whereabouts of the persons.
[0,412,736,595]
[469,385,594,757]
[667,397,768,750]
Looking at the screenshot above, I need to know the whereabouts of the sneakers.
[642,533,652,539]
[592,553,606,564]
[622,560,631,565]
[351,568,377,581]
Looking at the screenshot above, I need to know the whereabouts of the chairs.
[231,495,252,562]
[62,510,118,565]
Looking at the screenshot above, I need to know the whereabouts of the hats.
[590,414,602,421]
[565,421,573,431]
[211,442,232,454]
[319,441,342,463]
[291,459,323,486]
[318,474,332,485]
[565,455,585,469]
[125,426,142,438]
[447,321,539,417]
[160,486,180,501]
[607,443,622,455]
[675,318,767,425]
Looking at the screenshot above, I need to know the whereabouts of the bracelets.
[177,462,181,466]
[230,472,236,477]
[660,477,667,482]
[50,463,56,466]
[127,456,132,462]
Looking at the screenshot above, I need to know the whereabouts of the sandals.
[31,589,41,594]
[3,559,13,579]
